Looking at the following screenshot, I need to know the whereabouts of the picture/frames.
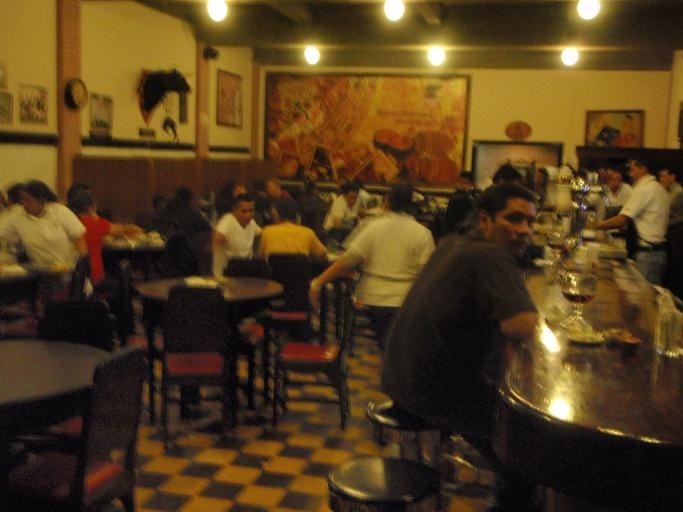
[585,110,645,146]
[264,70,472,188]
[471,140,564,189]
[217,68,243,129]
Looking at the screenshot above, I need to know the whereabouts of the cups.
[656,311,682,358]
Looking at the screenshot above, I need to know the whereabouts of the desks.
[497,210,683,454]
[0,336,113,434]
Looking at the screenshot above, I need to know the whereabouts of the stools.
[329,455,440,512]
[366,398,441,462]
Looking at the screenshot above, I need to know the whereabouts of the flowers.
[573,173,587,212]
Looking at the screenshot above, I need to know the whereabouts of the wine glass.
[542,230,565,276]
[559,257,598,333]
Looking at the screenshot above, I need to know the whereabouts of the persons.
[379,180,544,512]
[1,155,683,346]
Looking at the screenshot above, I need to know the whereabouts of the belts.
[638,243,667,252]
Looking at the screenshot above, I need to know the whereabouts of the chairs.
[11,349,148,512]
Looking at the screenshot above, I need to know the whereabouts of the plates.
[568,332,604,344]
